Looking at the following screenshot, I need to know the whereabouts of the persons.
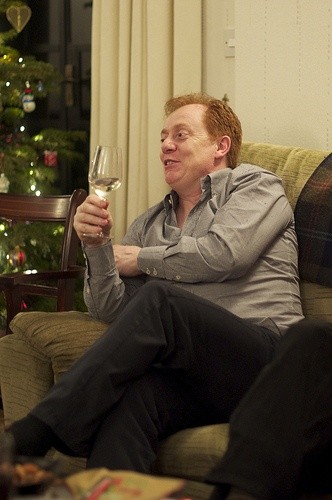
[0,93,306,476]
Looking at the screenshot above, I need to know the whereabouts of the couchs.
[0,142,332,500]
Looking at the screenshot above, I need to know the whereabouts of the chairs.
[0,185,87,336]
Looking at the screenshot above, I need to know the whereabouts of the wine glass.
[80,143,123,241]
